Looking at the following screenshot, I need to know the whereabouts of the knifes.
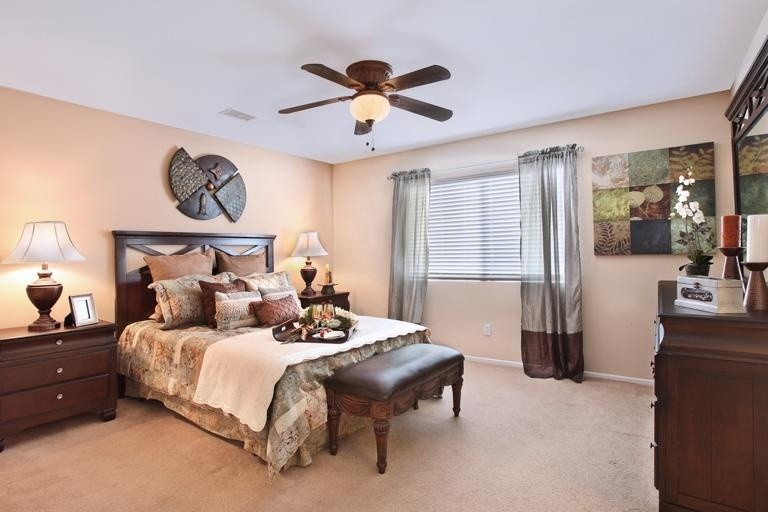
[280,333,303,344]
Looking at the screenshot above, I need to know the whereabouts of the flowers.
[666,166,717,274]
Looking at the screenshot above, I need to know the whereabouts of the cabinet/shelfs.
[648,279,767,511]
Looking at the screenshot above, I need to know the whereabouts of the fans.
[278,57,454,135]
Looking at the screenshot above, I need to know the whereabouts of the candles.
[323,264,332,284]
[745,214,767,263]
[720,214,740,247]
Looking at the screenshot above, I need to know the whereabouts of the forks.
[348,327,358,341]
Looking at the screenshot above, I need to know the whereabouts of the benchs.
[325,342,467,474]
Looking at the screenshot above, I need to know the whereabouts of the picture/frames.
[64,293,98,329]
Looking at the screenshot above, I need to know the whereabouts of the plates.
[312,330,346,340]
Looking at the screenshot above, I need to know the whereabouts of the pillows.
[258,286,300,308]
[249,295,300,327]
[198,277,245,330]
[147,273,229,330]
[241,270,291,294]
[215,248,267,277]
[144,248,214,323]
[213,288,265,331]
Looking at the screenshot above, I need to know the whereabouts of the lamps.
[289,232,327,296]
[2,221,86,333]
[346,93,392,150]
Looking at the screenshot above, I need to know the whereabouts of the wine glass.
[311,304,323,333]
[322,303,336,333]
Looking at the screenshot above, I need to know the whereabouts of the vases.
[686,264,708,277]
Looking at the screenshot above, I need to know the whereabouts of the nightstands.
[1,321,120,454]
[296,290,349,312]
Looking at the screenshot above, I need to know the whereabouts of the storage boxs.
[673,277,746,313]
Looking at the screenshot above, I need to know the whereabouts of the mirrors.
[724,35,767,261]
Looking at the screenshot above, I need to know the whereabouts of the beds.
[110,229,432,474]
